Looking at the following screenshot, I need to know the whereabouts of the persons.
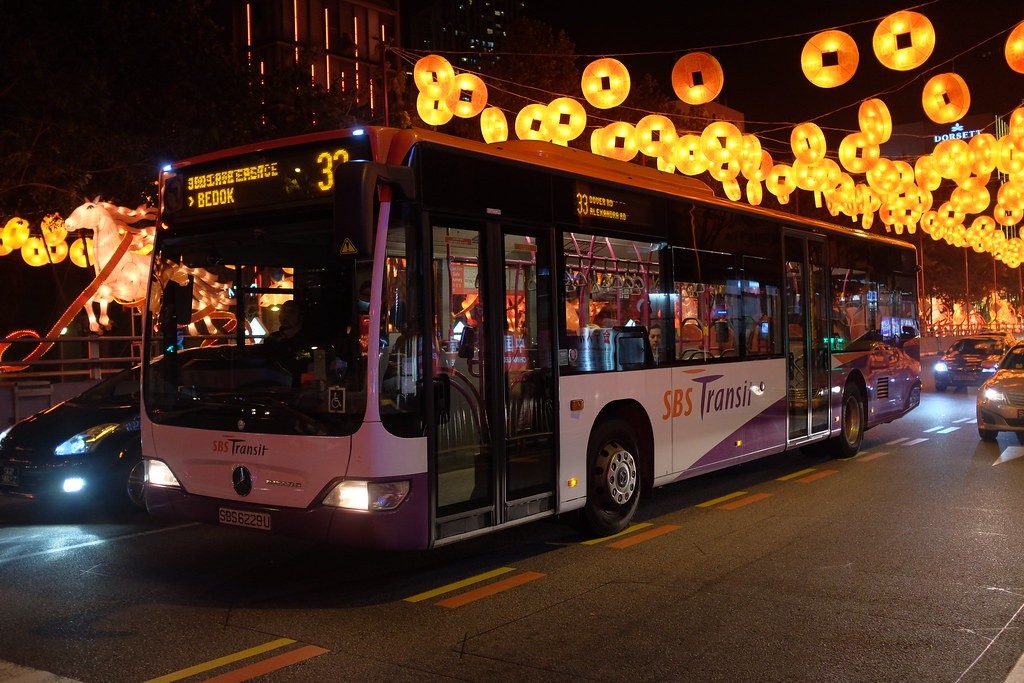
[264,301,311,358]
[567,291,663,365]
[385,304,440,394]
[987,344,994,355]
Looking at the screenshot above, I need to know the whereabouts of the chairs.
[678,304,883,359]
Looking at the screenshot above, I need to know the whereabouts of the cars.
[0,344,263,524]
[933,335,1017,393]
[976,339,1024,441]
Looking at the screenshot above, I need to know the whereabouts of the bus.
[136,125,923,555]
